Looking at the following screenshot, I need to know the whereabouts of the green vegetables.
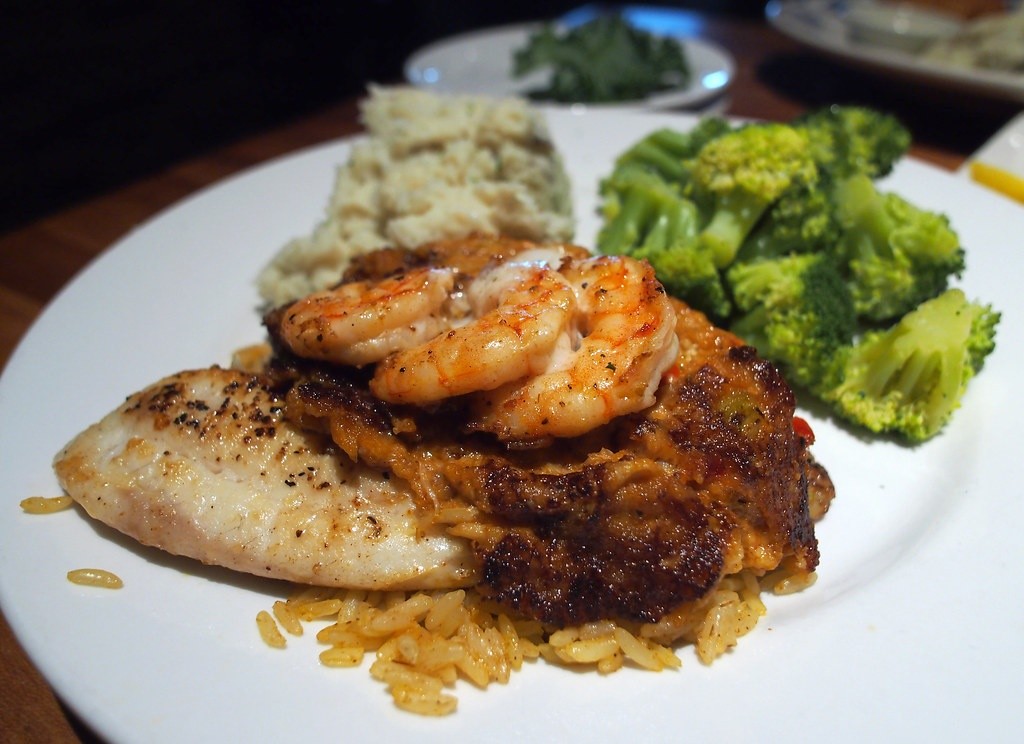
[512,11,688,102]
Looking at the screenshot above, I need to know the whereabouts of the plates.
[404,24,736,108]
[1,103,1024,744]
[761,0,1024,102]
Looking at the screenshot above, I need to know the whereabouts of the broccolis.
[591,104,1000,447]
